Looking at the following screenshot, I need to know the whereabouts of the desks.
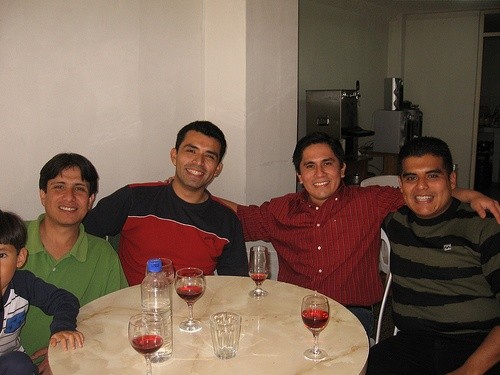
[48,276,370,375]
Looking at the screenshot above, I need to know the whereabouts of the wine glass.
[174,267,207,333]
[128,312,165,375]
[301,294,330,362]
[249,245,269,296]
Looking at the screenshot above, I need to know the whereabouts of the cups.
[210,311,241,359]
[145,257,174,284]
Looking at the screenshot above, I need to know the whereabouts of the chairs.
[366,227,392,348]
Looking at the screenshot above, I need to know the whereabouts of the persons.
[0,152,130,375]
[366,136,500,375]
[83,120,249,288]
[164,132,500,325]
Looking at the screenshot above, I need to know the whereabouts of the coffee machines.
[306,80,375,186]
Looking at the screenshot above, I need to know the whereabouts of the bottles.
[141,259,173,362]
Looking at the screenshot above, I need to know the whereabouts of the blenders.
[373,77,423,156]
